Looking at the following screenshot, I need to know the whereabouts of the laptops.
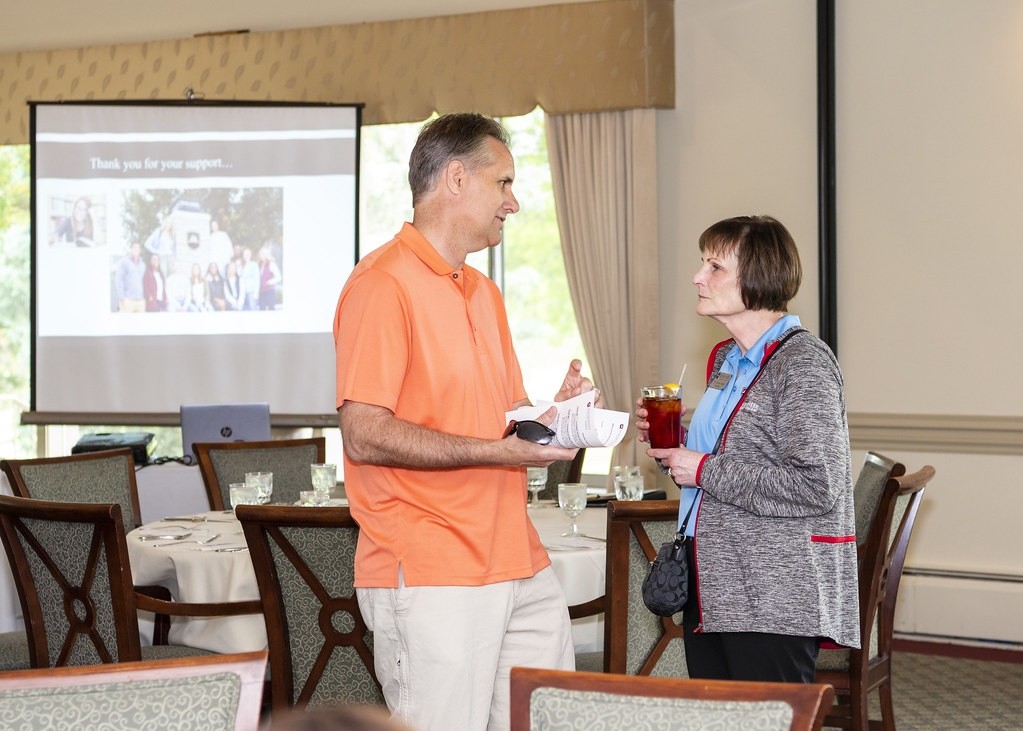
[180,403,271,465]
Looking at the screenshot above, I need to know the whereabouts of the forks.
[153,533,222,547]
[139,523,205,531]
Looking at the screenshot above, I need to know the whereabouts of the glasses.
[502,420,555,445]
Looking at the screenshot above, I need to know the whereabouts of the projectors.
[71,432,157,464]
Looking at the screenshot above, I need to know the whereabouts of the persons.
[636,215,861,684]
[52,197,93,247]
[333,113,606,731]
[117,218,282,313]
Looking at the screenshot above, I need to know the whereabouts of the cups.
[228,483,260,515]
[310,464,337,493]
[244,472,272,503]
[640,386,683,462]
[299,490,330,506]
[613,466,644,501]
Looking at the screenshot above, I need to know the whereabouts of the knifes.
[164,516,233,523]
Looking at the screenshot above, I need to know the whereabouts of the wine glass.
[526,466,548,508]
[558,483,586,538]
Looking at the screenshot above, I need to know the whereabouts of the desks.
[125,500,607,658]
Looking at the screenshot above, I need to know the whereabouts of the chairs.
[0,437,935,731]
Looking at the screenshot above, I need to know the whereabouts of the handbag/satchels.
[642,540,689,617]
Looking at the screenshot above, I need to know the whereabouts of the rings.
[593,387,601,393]
[668,468,672,474]
